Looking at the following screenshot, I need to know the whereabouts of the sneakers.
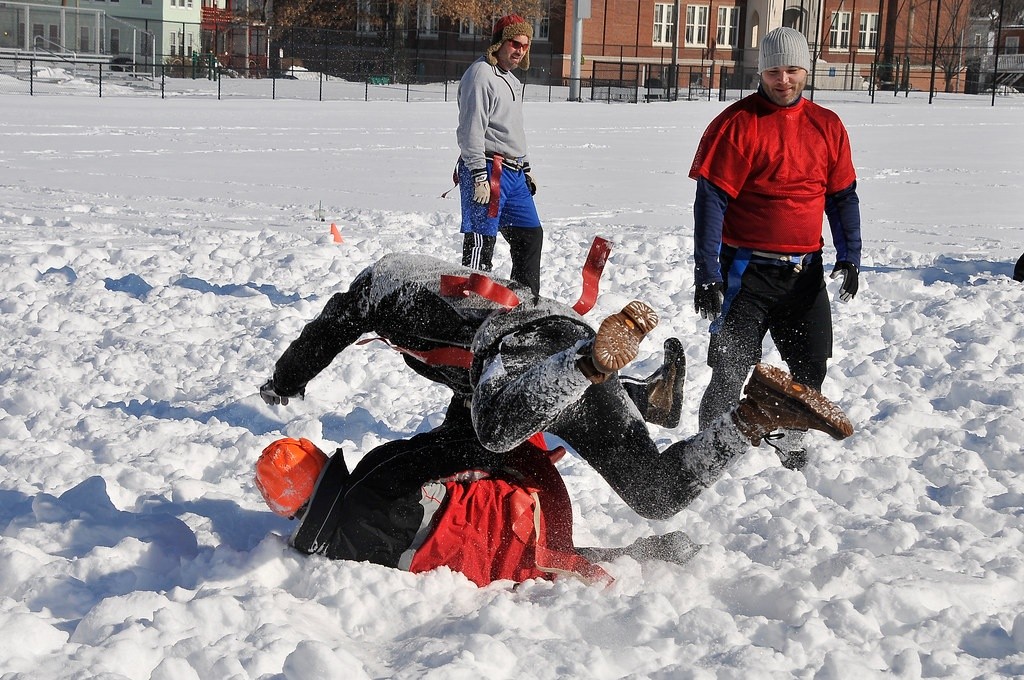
[645,338,685,429]
[772,423,806,469]
[591,301,657,373]
[744,363,852,439]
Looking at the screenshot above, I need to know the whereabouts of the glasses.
[507,38,529,52]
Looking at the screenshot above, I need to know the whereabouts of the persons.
[455,12,544,306]
[253,252,854,588]
[686,26,862,431]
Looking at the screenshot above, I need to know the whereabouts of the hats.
[253,437,329,517]
[758,27,810,75]
[486,15,533,70]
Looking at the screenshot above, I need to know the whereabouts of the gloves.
[470,167,490,205]
[694,281,722,321]
[830,261,858,302]
[260,379,289,406]
[525,174,536,196]
